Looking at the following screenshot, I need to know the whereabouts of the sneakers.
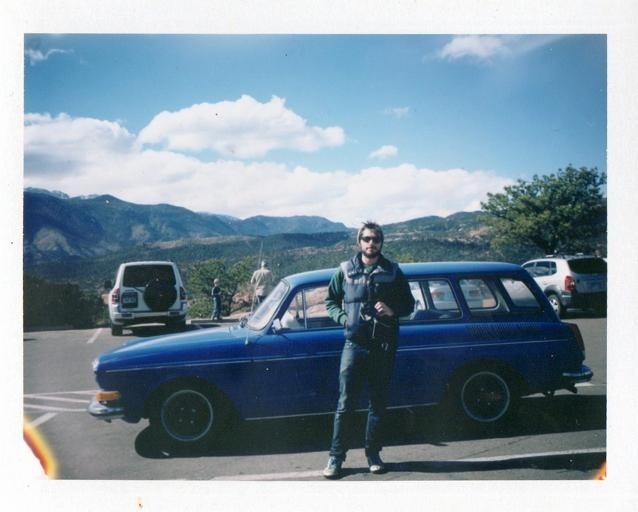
[321,454,347,478]
[365,451,386,473]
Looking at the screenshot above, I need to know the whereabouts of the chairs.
[411,309,455,320]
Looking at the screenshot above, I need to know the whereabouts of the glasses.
[359,235,383,244]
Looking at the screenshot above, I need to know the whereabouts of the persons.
[251,261,274,304]
[209,278,224,321]
[322,220,415,479]
